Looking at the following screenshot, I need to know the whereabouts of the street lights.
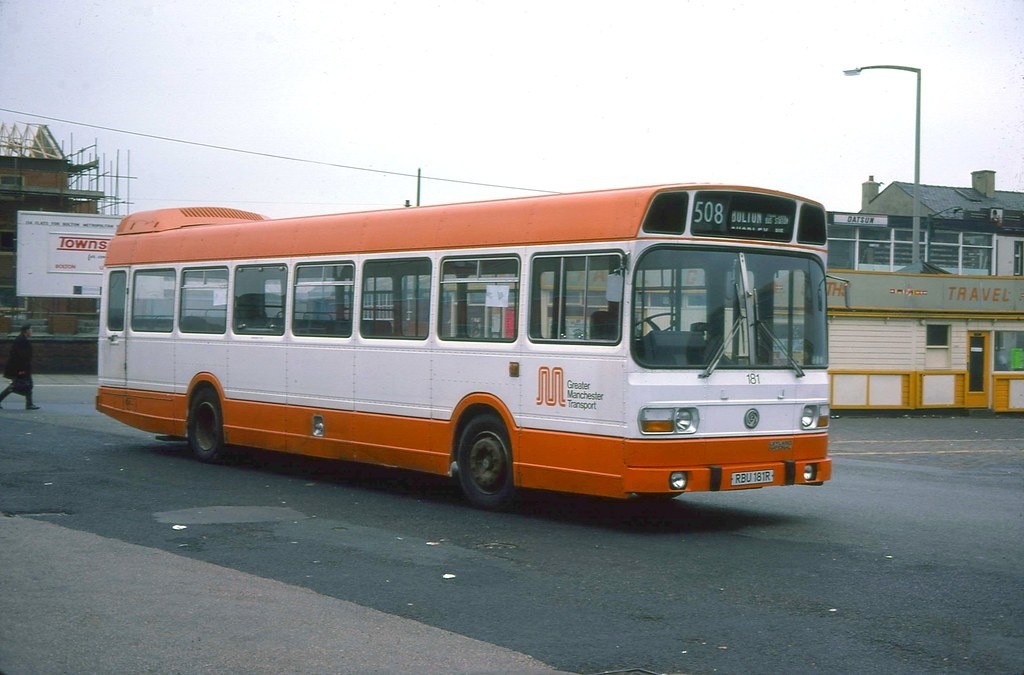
[833,56,924,266]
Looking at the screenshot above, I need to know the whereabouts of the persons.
[0,321,42,410]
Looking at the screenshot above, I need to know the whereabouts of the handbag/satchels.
[12,374,32,395]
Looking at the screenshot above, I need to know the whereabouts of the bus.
[87,178,840,516]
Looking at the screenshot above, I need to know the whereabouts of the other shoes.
[26,404,41,409]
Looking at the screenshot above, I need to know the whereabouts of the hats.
[21,323,31,330]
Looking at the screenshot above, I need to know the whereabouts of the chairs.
[589,311,617,340]
[106,293,589,339]
